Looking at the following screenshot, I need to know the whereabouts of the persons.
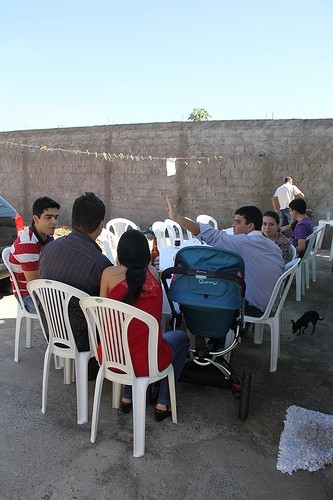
[97,229,190,421]
[271,177,305,238]
[261,210,292,265]
[162,191,287,351]
[8,196,61,314]
[279,198,315,259]
[38,191,115,382]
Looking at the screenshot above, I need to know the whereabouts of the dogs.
[291,310,326,335]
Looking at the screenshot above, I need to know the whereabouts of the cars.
[0,195,24,279]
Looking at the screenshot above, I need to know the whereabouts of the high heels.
[121,399,133,413]
[154,405,171,421]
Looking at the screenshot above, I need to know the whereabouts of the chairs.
[2,214,333,456]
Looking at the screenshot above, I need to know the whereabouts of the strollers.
[161,246,252,421]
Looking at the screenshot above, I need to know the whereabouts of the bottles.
[151,238,159,270]
